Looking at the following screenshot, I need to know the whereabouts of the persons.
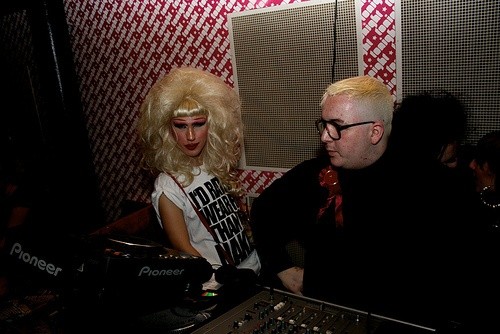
[250,76,500,334]
[136,67,261,277]
[392,94,500,194]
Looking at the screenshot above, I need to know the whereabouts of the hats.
[469,132,500,161]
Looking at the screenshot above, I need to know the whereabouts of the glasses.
[315,119,375,141]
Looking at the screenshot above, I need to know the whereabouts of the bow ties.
[316,166,343,227]
